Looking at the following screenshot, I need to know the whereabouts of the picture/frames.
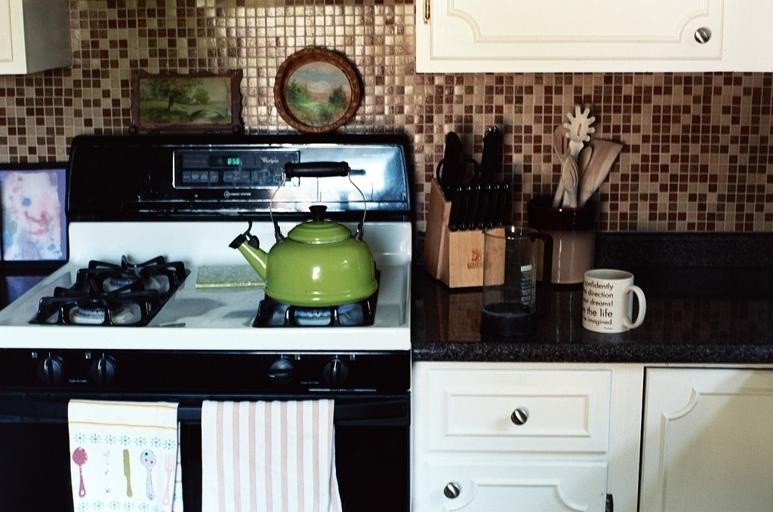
[129,69,245,132]
[0,162,69,267]
[273,47,361,134]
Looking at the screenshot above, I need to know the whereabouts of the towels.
[68,398,185,512]
[201,399,342,511]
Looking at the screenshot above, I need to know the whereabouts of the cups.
[479,226,555,320]
[528,193,598,286]
[580,267,646,337]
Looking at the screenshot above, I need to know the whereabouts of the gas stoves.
[0,253,413,394]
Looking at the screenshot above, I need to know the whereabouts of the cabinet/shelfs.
[410,362,612,512]
[415,2,771,73]
[611,366,773,511]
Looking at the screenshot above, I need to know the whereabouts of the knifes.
[443,124,510,232]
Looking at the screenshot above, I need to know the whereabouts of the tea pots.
[227,159,381,309]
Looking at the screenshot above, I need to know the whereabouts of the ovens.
[0,395,411,511]
